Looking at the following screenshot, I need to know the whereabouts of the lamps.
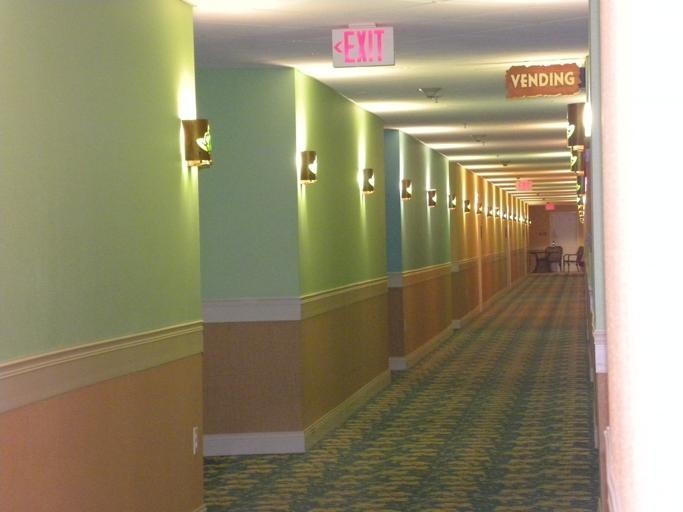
[402,179,413,200]
[183,119,213,169]
[363,169,375,194]
[567,103,585,224]
[300,151,317,184]
[428,189,437,207]
[449,193,532,225]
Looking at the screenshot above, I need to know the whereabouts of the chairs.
[545,246,563,272]
[563,247,584,272]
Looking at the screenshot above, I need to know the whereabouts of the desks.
[528,250,554,273]
